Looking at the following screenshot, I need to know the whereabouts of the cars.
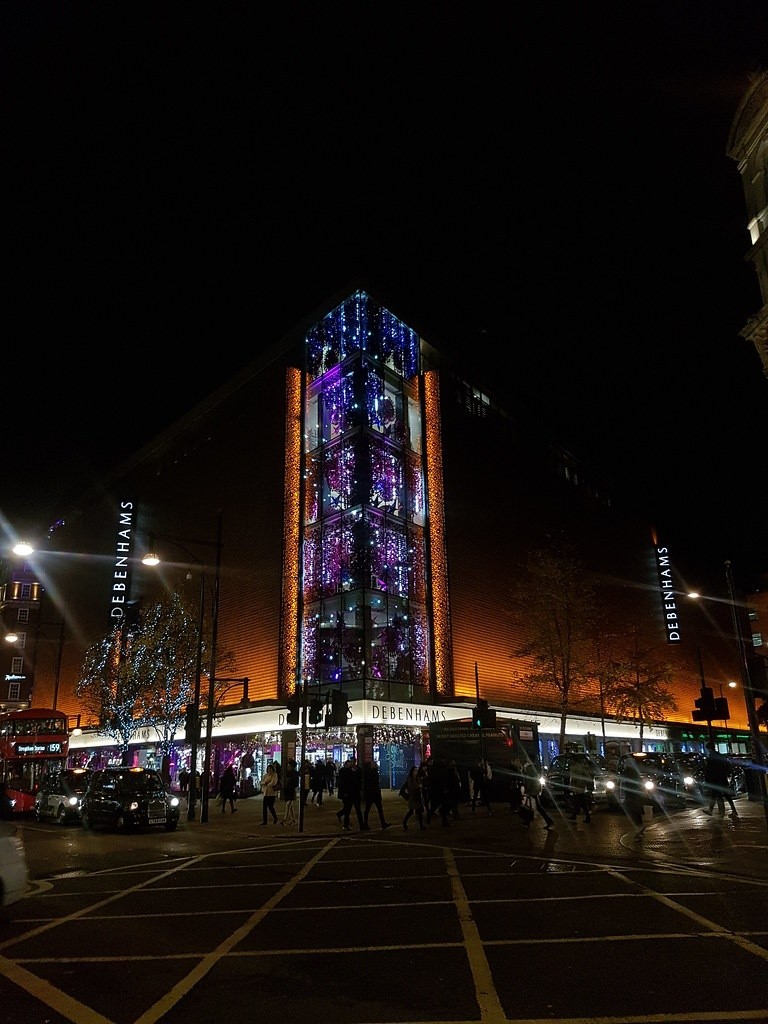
[33,765,95,822]
[545,753,747,811]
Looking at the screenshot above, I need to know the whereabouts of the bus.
[0,708,67,815]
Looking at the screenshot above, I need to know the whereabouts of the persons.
[156,740,739,833]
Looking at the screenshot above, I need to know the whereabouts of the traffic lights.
[185,704,197,746]
[308,700,324,724]
[285,699,300,724]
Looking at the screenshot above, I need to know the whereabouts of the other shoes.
[702,809,713,816]
[260,822,267,825]
[316,802,324,808]
[274,817,278,825]
[336,813,342,824]
[442,823,454,828]
[281,820,286,825]
[382,823,391,829]
[402,821,408,830]
[232,808,238,813]
[568,816,576,820]
[289,820,296,826]
[222,811,226,813]
[544,822,554,830]
[729,812,739,817]
[364,826,371,830]
[420,826,427,831]
[583,818,592,824]
[343,826,352,831]
[521,827,528,832]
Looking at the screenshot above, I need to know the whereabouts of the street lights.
[201,683,245,822]
[719,682,736,753]
[141,553,222,825]
[687,590,768,825]
[473,703,497,728]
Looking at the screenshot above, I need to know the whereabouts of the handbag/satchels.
[400,782,411,801]
[519,797,535,822]
[214,793,222,806]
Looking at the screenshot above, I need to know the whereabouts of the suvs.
[82,765,185,837]
